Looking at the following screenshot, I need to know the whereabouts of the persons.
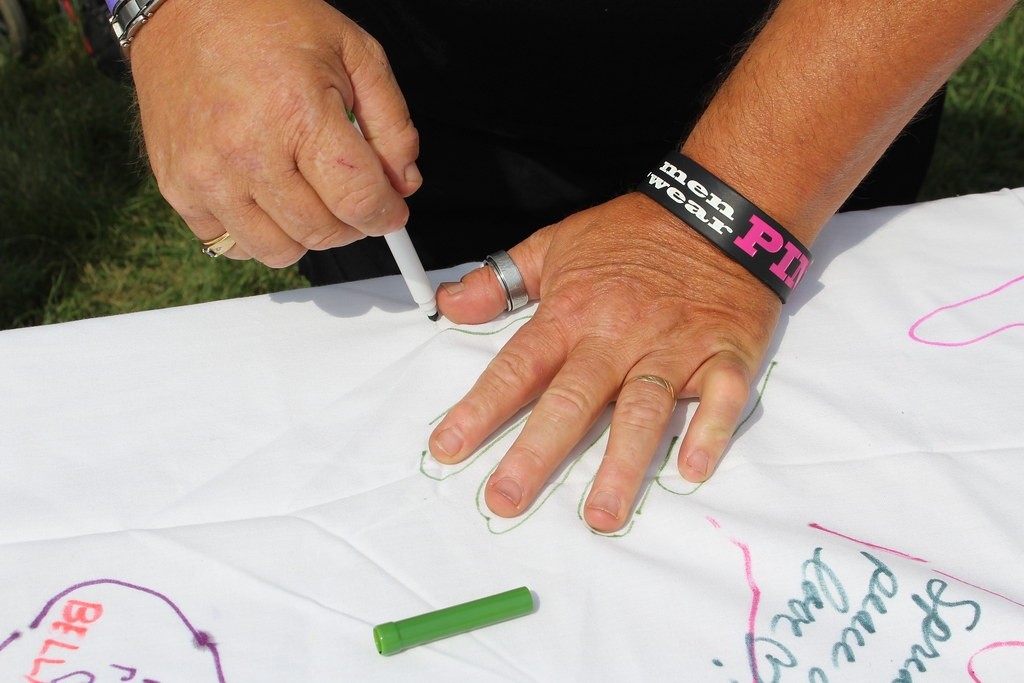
[107,0,1014,533]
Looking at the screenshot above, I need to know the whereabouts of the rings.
[484,251,529,312]
[626,374,677,410]
[201,233,235,258]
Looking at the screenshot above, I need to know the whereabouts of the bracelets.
[108,0,165,57]
[641,154,813,303]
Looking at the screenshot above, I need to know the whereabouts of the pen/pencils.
[345,107,438,322]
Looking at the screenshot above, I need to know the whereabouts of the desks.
[2,181,1019,681]
